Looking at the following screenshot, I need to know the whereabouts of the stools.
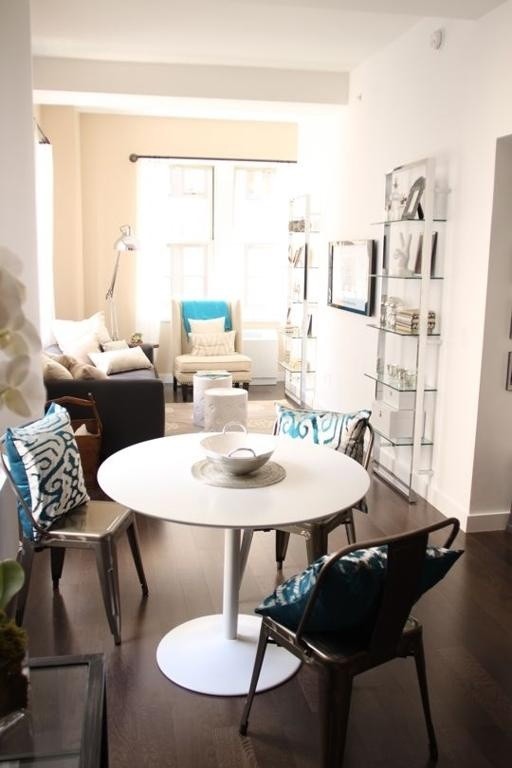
[190,369,233,428]
[205,387,250,432]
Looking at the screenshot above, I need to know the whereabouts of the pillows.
[187,315,225,334]
[250,542,466,638]
[4,398,93,539]
[184,327,238,357]
[42,309,153,381]
[266,403,370,457]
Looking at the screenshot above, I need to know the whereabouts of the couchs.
[43,325,168,493]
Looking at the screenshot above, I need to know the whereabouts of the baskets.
[44,393,103,477]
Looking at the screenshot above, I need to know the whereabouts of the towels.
[180,298,232,330]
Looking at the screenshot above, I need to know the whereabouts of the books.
[395,308,436,334]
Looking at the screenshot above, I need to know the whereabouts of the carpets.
[165,395,297,437]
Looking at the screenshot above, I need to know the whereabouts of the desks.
[93,427,372,701]
[0,646,114,765]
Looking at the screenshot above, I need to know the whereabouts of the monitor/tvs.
[327,239,375,316]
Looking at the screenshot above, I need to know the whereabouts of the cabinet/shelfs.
[239,327,282,387]
[278,191,321,409]
[358,153,446,508]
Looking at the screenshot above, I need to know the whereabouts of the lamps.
[105,221,138,339]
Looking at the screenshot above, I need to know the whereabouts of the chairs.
[236,396,375,569]
[2,411,153,645]
[238,508,470,768]
[170,296,254,403]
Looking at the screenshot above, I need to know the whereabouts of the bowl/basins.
[196,421,276,477]
